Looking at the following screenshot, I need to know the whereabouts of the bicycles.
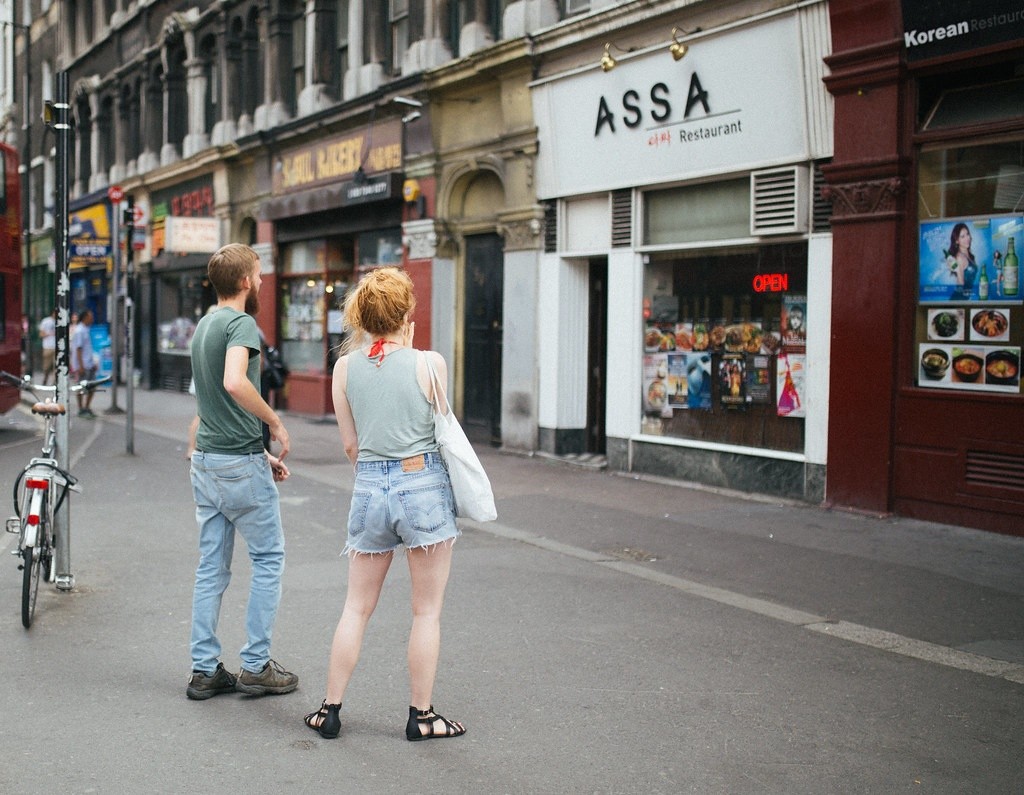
[0,370,114,630]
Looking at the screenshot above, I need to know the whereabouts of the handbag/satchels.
[263,343,288,389]
[425,351,497,521]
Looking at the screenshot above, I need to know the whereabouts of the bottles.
[943,249,958,269]
[1004,237,1018,295]
[979,264,989,299]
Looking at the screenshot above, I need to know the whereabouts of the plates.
[972,311,1008,336]
[933,312,958,337]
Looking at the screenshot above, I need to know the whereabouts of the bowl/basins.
[952,354,985,381]
[986,351,1018,384]
[921,348,950,380]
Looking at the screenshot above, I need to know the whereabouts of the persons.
[69,313,78,339]
[188,322,281,456]
[942,223,978,301]
[37,308,56,389]
[718,360,744,398]
[992,249,1004,296]
[69,310,99,417]
[184,237,300,700]
[783,306,806,342]
[302,270,467,742]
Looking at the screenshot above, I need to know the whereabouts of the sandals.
[305,699,342,739]
[406,705,465,740]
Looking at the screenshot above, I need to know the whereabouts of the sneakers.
[187,669,238,700]
[234,665,298,695]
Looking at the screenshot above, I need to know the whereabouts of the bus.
[0,142,23,415]
